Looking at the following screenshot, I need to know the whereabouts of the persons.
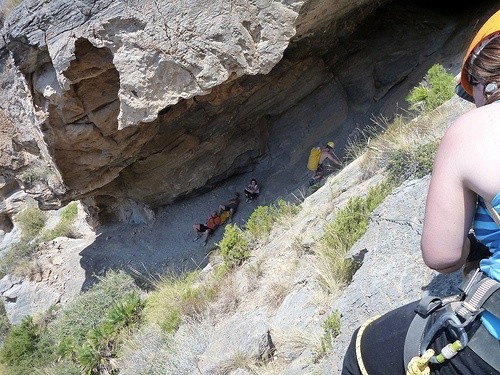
[341,10,500,375]
[244,179,259,202]
[309,141,344,186]
[193,193,241,247]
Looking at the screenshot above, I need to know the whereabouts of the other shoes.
[202,239,208,247]
[247,199,253,203]
[193,235,201,241]
[229,216,233,222]
[309,178,315,187]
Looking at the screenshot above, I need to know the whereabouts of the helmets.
[327,141,335,150]
[460,9,500,97]
